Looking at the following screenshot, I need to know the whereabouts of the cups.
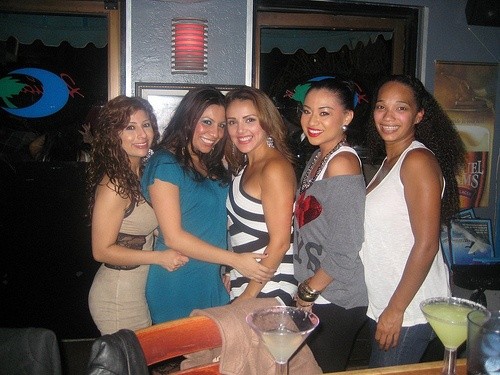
[467,308,500,375]
[441,108,490,209]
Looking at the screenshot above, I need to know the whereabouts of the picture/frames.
[134,80,239,170]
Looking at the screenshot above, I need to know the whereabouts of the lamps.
[171,16,209,75]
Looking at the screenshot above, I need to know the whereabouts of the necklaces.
[298,135,347,193]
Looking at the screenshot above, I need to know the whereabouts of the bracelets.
[297,281,319,307]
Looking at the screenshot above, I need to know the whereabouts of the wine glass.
[419,296,491,375]
[246,306,320,374]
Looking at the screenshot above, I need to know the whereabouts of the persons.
[87,95,187,336]
[146,85,277,325]
[223,86,298,309]
[362,75,468,367]
[292,77,367,373]
